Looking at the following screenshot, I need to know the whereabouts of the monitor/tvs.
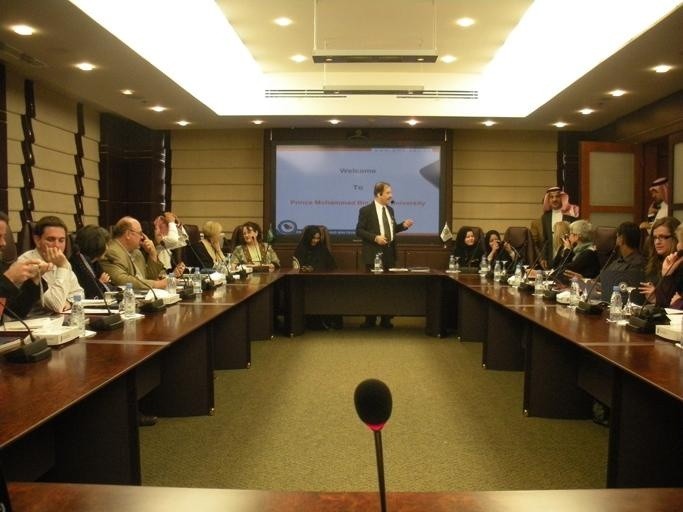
[269,139,448,243]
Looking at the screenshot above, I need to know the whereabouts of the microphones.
[485,240,511,278]
[223,237,246,280]
[543,242,577,301]
[354,378,392,512]
[72,243,124,331]
[391,200,395,204]
[0,301,52,364]
[575,245,620,314]
[211,243,235,283]
[499,241,527,285]
[518,240,549,293]
[186,240,216,290]
[626,249,683,333]
[463,236,481,273]
[108,258,167,313]
[160,240,196,300]
[252,236,269,273]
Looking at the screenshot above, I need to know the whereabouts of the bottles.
[494,261,500,283]
[514,265,521,287]
[481,255,488,277]
[569,278,579,308]
[609,285,622,320]
[534,270,544,297]
[124,283,135,316]
[375,254,381,272]
[448,254,455,272]
[192,266,202,294]
[167,273,176,294]
[70,296,85,337]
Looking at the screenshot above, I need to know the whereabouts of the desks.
[270,244,456,272]
[254,264,458,337]
[447,269,683,485]
[0,264,286,485]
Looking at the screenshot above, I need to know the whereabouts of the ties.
[381,206,393,244]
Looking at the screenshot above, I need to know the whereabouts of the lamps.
[312,49,438,64]
[4,480,683,512]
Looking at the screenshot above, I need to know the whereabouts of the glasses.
[652,233,673,241]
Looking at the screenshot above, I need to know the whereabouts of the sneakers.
[591,413,609,426]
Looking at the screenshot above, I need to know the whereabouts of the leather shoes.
[378,320,396,330]
[359,321,376,329]
[138,414,158,425]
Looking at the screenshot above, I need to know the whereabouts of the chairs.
[231,226,246,252]
[504,227,534,267]
[141,222,155,241]
[184,224,213,266]
[471,227,484,250]
[68,233,77,257]
[22,219,37,254]
[594,226,619,262]
[298,226,331,253]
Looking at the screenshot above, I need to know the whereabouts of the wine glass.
[224,253,232,266]
[623,287,636,312]
[501,261,508,282]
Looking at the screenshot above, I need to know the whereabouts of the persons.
[449,178,682,427]
[294,226,343,329]
[0,211,279,427]
[356,182,414,329]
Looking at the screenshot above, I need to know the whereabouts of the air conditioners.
[263,88,346,99]
[395,90,480,100]
[324,84,425,94]
[0,38,49,70]
